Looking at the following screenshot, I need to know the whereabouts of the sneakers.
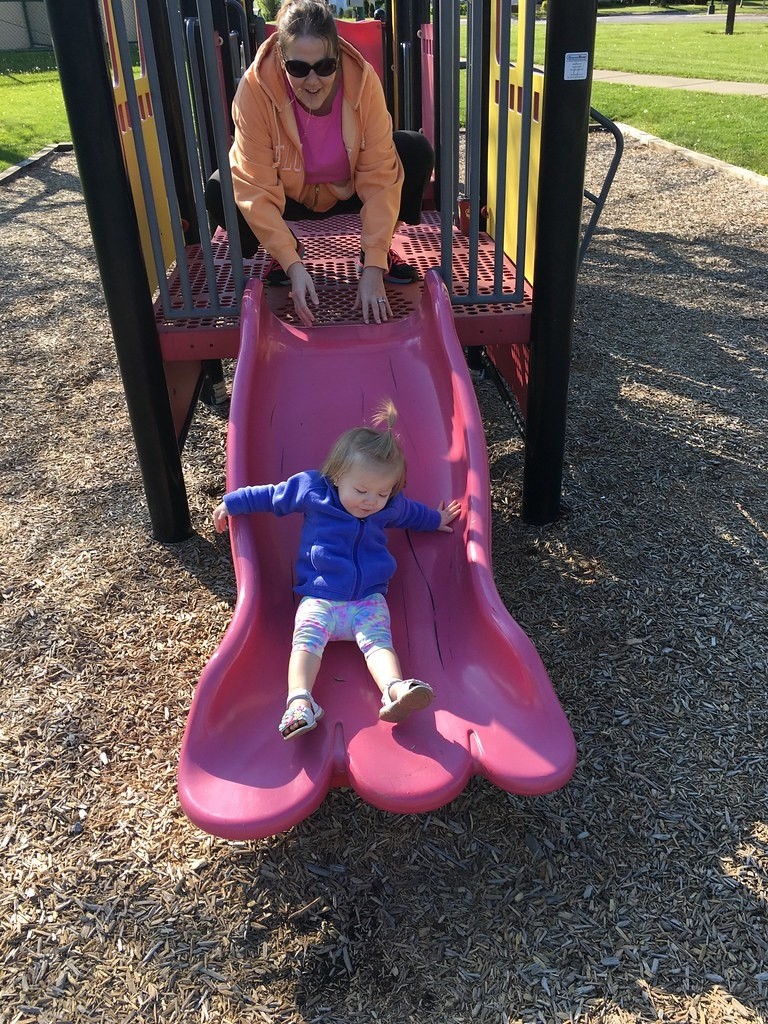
[263,239,305,287]
[356,248,419,284]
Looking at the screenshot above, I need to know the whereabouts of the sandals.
[279,690,324,742]
[379,678,435,723]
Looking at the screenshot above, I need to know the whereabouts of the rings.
[378,297,386,302]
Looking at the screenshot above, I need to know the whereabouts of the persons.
[204,0,434,328]
[213,400,461,741]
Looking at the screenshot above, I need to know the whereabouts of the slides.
[176,269,577,838]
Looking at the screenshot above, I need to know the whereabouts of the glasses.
[279,45,339,79]
